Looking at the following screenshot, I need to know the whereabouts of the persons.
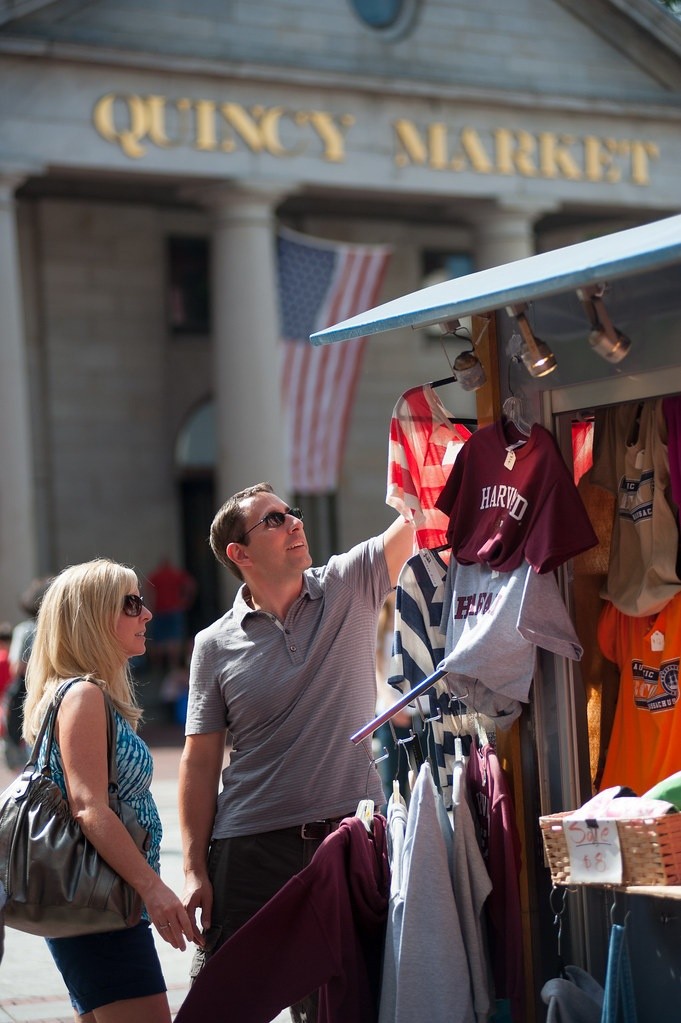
[0,578,53,772]
[18,556,196,1023]
[146,545,205,740]
[370,588,422,828]
[178,483,420,1023]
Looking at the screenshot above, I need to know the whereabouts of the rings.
[160,924,170,930]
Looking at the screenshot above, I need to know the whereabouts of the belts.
[274,820,361,840]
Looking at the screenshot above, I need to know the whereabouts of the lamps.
[575,283,632,365]
[439,319,489,392]
[504,302,559,380]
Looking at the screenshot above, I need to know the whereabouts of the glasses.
[115,595,148,616]
[237,509,305,543]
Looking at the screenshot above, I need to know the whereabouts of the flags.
[266,221,394,495]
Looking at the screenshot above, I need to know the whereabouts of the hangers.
[308,377,678,1023]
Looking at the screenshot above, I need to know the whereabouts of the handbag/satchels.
[0,677,148,937]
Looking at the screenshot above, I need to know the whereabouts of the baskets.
[537,806,681,884]
